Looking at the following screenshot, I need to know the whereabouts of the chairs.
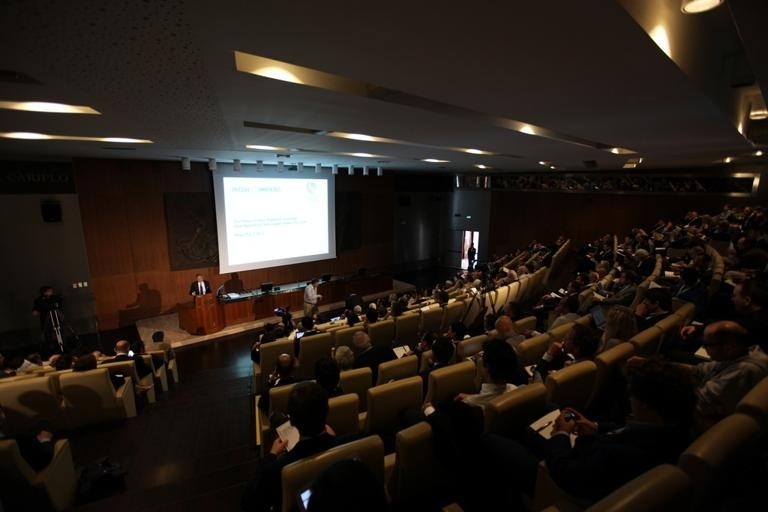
[1,438,79,510]
[1,345,178,432]
[252,235,768,511]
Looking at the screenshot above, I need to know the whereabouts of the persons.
[248,204,768,512]
[2,284,175,511]
[187,272,212,296]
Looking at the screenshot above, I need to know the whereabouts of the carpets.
[199,282,202,295]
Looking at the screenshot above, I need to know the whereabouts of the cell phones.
[562,412,577,419]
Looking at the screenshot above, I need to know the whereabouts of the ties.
[261,283,273,295]
[216,285,225,303]
[322,274,331,283]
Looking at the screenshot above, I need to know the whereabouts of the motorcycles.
[39,309,84,354]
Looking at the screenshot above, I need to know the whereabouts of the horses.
[299,487,312,510]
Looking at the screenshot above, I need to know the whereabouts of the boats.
[397,194,411,207]
[40,199,62,222]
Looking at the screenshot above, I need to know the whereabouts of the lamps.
[1,345,178,432]
[1,438,79,510]
[748,94,768,123]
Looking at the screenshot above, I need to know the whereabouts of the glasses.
[748,94,768,123]
